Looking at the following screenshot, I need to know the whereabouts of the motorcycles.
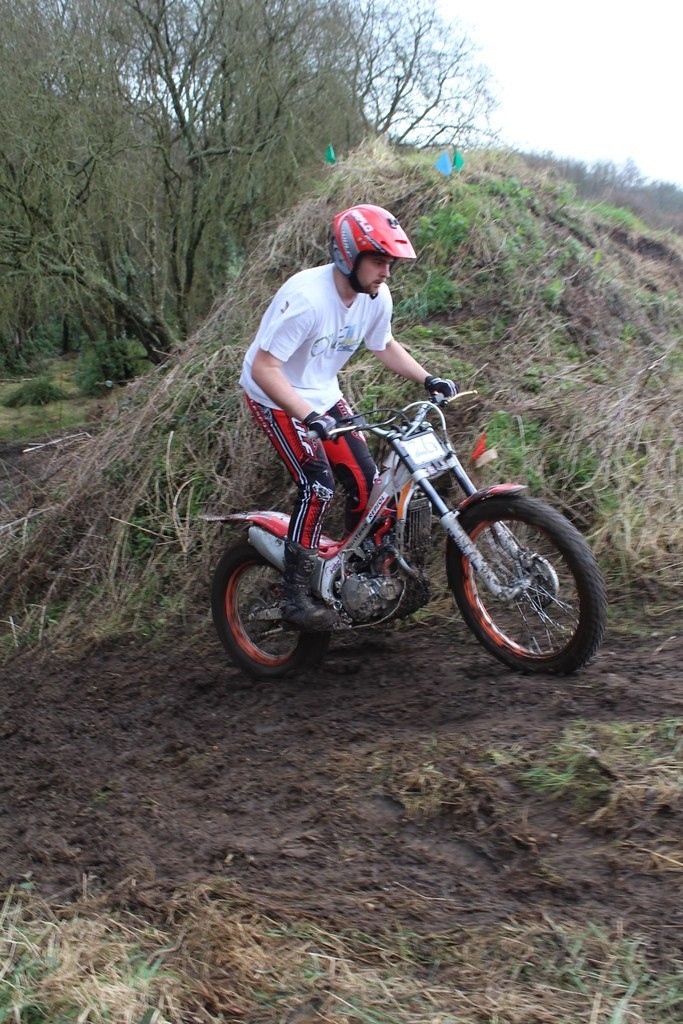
[198,386,609,684]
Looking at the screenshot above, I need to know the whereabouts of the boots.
[279,541,338,632]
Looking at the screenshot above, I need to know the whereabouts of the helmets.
[328,204,418,276]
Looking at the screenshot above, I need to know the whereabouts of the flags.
[435,150,453,175]
[452,150,465,172]
[325,142,336,164]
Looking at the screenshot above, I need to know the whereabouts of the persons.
[233,201,458,633]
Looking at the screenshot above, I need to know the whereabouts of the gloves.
[302,411,341,444]
[425,375,459,398]
